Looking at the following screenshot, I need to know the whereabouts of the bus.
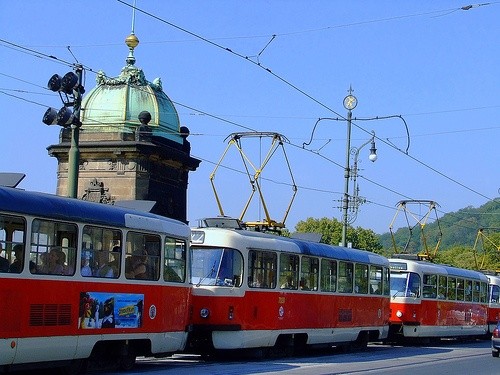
[0,173,500,366]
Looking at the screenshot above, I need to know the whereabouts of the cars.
[492,320,500,357]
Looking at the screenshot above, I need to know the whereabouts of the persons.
[309,273,339,291]
[8,237,154,279]
[250,273,267,288]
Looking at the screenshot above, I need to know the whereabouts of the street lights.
[340,131,378,249]
[42,63,86,199]
